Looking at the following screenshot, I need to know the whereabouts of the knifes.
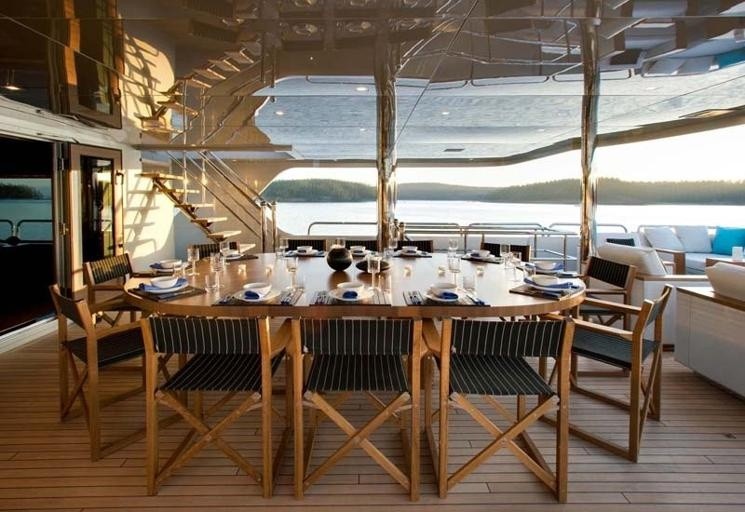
[527,288,558,298]
[314,290,326,304]
[157,289,193,299]
[466,293,480,306]
[281,288,296,303]
[407,290,420,305]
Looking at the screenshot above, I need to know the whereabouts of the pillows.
[675,225,712,252]
[644,227,683,256]
[712,226,745,255]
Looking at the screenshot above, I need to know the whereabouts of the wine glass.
[447,239,462,291]
[186,239,231,298]
[367,256,382,291]
[284,256,304,292]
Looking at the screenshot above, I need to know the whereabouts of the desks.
[121,248,589,415]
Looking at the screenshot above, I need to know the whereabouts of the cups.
[732,246,744,262]
[463,272,476,291]
[384,248,394,262]
[499,242,536,280]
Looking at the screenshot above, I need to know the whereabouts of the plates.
[233,288,281,303]
[399,250,423,256]
[229,253,245,260]
[532,266,559,274]
[329,288,374,302]
[348,249,372,255]
[144,281,189,294]
[523,277,573,292]
[421,289,466,302]
[295,250,317,256]
[467,253,494,260]
[148,261,188,272]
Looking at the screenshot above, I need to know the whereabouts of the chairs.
[51,283,191,464]
[479,241,533,263]
[81,252,150,326]
[544,255,638,386]
[536,282,672,466]
[343,239,380,252]
[137,313,293,500]
[590,242,710,346]
[190,241,239,260]
[288,314,423,501]
[420,316,577,503]
[393,239,435,253]
[675,263,745,396]
[285,239,328,251]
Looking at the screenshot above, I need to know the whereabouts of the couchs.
[642,222,745,272]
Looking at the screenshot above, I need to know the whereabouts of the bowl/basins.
[336,281,365,297]
[228,249,237,256]
[160,258,182,268]
[472,249,490,257]
[533,274,558,286]
[242,282,273,296]
[150,275,179,289]
[350,245,365,252]
[296,246,313,252]
[430,282,458,296]
[402,246,417,253]
[534,259,556,271]
[356,259,391,274]
